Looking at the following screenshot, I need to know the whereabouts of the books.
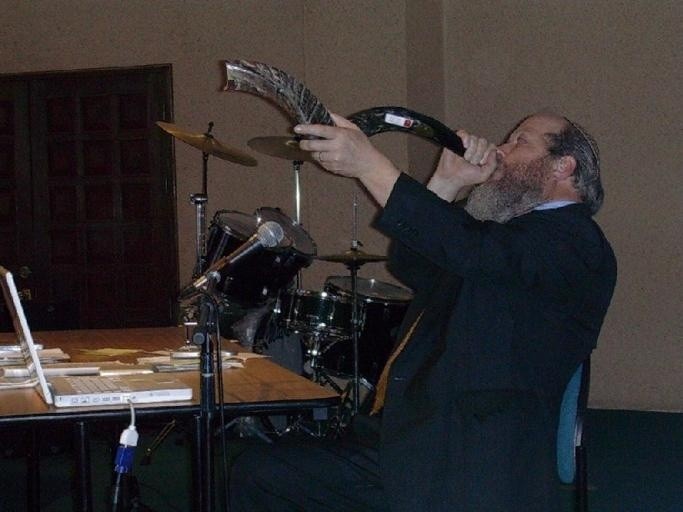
[0,343,244,377]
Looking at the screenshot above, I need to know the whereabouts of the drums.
[310,275,413,380]
[253,206,317,308]
[283,290,365,340]
[204,209,292,308]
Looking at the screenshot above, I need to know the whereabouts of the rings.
[318,151,323,162]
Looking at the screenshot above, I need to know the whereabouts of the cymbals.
[156,121,259,166]
[248,136,317,162]
[313,250,393,265]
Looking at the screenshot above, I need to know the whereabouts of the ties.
[368,308,427,416]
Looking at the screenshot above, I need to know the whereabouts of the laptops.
[0,265,193,409]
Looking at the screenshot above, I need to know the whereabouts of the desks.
[1,326,339,511]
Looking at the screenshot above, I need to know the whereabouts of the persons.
[228,110,617,511]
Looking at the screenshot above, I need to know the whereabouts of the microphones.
[177,220,285,300]
